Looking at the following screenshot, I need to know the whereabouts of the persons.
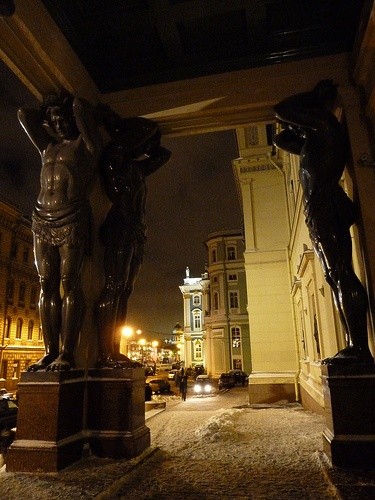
[92,103,174,368]
[145,383,152,401]
[197,367,202,375]
[16,90,104,373]
[152,360,156,375]
[173,371,188,401]
[272,119,375,366]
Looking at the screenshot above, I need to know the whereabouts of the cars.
[220,370,249,388]
[149,378,170,394]
[145,361,156,375]
[0,399,18,437]
[167,369,180,379]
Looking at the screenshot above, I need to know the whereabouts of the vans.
[195,375,210,394]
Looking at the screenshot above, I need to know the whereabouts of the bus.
[160,364,172,371]
